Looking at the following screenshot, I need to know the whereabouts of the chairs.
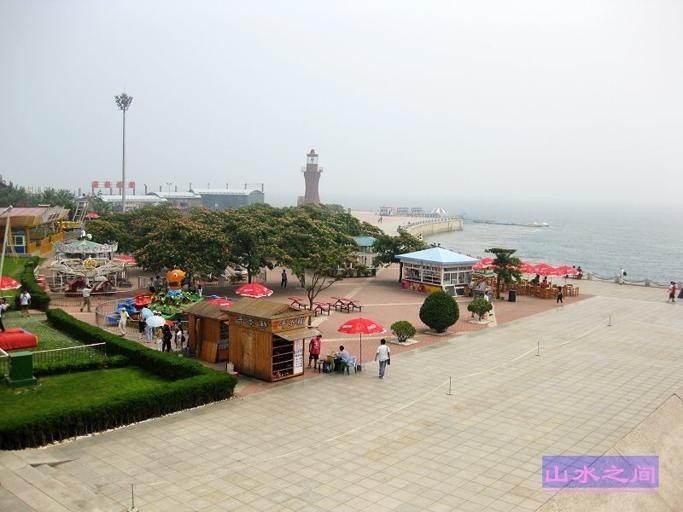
[490,275,579,301]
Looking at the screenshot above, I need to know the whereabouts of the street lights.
[113,91,132,213]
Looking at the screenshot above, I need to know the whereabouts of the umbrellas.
[471,258,580,287]
[234,284,273,300]
[337,318,387,365]
[0,276,22,291]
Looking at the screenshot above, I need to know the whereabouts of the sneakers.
[379,375,383,379]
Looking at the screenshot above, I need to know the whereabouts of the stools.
[316,351,356,375]
[289,296,365,317]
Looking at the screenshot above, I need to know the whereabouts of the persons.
[279,269,287,288]
[0,298,5,331]
[307,334,322,369]
[196,284,204,295]
[147,277,156,291]
[333,344,350,372]
[78,284,95,313]
[118,306,183,351]
[556,286,563,303]
[618,266,624,285]
[482,290,493,317]
[18,288,32,317]
[666,281,677,302]
[374,338,391,379]
[576,266,584,280]
[671,282,676,295]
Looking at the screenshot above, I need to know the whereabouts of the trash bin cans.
[509,290,516,302]
